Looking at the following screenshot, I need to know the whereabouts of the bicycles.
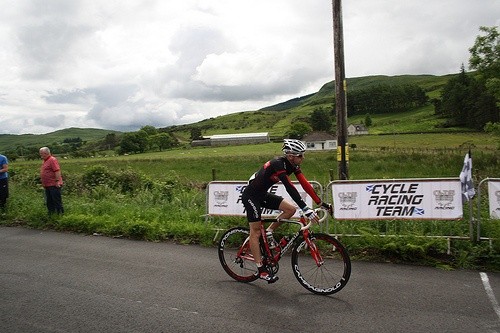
[218,200,351,295]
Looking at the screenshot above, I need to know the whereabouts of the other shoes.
[258,268,279,281]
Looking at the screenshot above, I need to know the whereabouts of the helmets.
[281,139,306,156]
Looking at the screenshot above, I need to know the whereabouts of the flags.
[459,151,476,202]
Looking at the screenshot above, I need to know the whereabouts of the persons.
[39,147,64,217]
[0,154,9,209]
[241,139,334,283]
